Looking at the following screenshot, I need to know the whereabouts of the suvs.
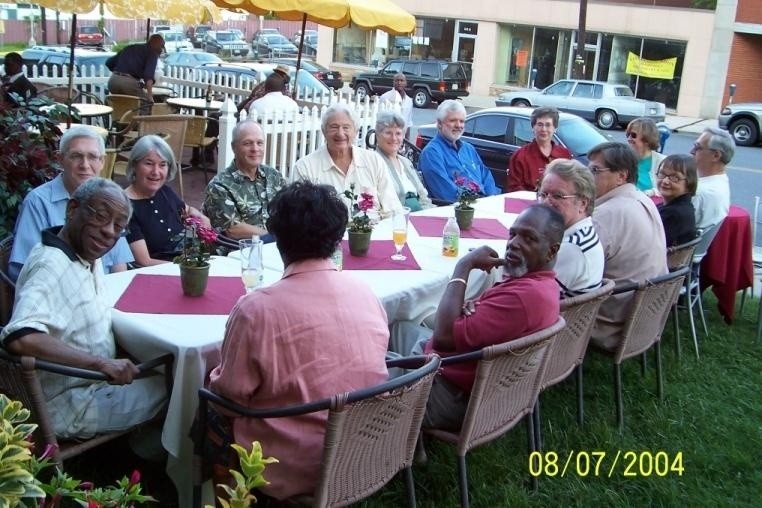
[348,58,471,106]
[185,60,361,130]
[69,25,105,48]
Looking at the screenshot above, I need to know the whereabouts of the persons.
[196,118,288,261]
[506,106,738,310]
[289,102,404,237]
[0,174,180,507]
[364,110,439,216]
[379,72,413,137]
[201,175,393,507]
[120,133,212,271]
[234,63,292,126]
[0,52,38,112]
[104,33,166,117]
[247,71,300,125]
[416,98,504,208]
[6,124,136,287]
[380,204,566,430]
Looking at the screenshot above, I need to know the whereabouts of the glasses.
[626,131,638,139]
[693,143,717,151]
[656,172,686,182]
[536,192,576,207]
[88,205,132,237]
[590,167,612,174]
[69,153,100,163]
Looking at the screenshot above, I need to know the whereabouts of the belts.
[113,71,132,77]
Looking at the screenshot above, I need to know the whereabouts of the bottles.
[332,243,343,271]
[441,216,460,257]
[206,85,212,102]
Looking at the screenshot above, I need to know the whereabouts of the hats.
[273,65,291,83]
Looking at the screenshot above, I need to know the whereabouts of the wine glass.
[238,239,264,292]
[390,206,411,261]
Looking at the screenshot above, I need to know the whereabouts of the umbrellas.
[1,0,225,130]
[207,0,417,99]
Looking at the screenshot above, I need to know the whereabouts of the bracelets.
[447,277,467,288]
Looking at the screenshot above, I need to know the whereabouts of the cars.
[719,96,761,145]
[164,52,223,74]
[274,57,345,92]
[415,78,669,190]
[17,39,122,79]
[148,23,320,59]
